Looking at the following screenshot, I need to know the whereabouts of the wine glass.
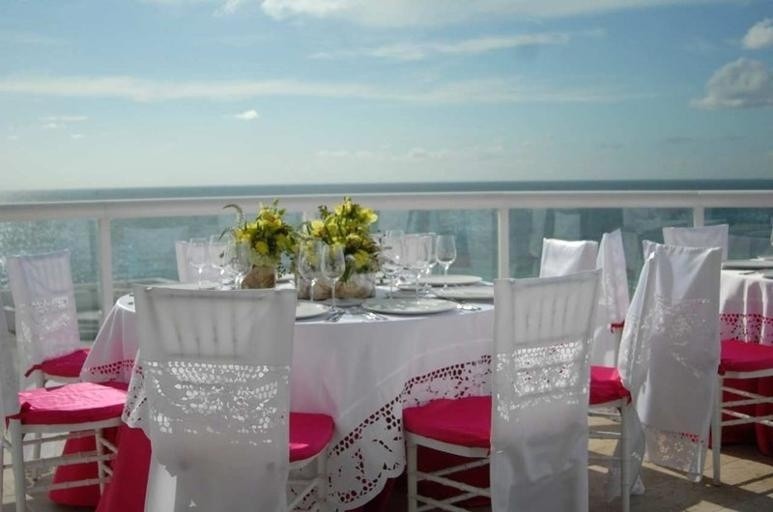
[185,234,254,289]
[298,242,346,315]
[369,232,456,301]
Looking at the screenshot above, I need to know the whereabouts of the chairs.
[6,227,772,512]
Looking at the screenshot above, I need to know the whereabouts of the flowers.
[295,196,383,279]
[219,197,304,277]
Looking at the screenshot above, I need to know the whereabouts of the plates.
[293,300,326,320]
[425,274,495,302]
[362,299,458,314]
